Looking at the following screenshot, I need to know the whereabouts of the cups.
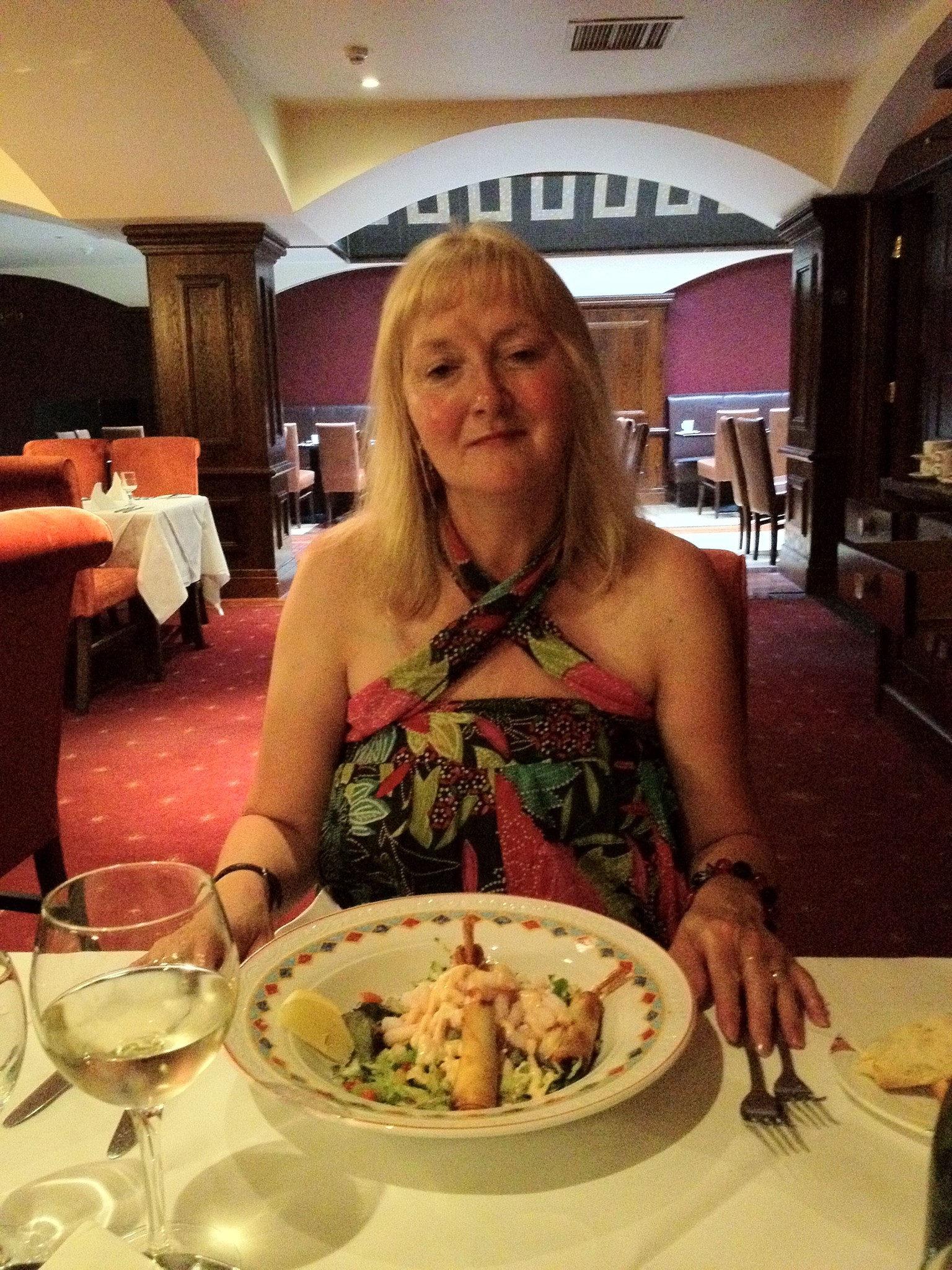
[920,439,952,476]
[682,419,693,432]
[0,950,28,1110]
[311,435,319,444]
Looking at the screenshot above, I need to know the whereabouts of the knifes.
[123,506,144,513]
[4,1070,71,1130]
[106,1107,140,1158]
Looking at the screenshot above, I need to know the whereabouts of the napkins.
[83,472,129,511]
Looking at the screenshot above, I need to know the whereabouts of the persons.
[129,221,832,1056]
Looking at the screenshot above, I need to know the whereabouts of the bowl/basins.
[224,892,697,1138]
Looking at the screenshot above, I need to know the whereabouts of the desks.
[81,497,231,686]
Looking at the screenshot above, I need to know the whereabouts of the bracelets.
[213,863,281,909]
[688,858,775,930]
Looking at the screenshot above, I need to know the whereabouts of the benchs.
[664,390,790,508]
[283,404,368,522]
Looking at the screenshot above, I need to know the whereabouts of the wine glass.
[121,472,139,499]
[28,861,241,1270]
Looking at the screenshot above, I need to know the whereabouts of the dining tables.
[0,953,952,1270]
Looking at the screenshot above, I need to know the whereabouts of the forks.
[740,1032,798,1127]
[772,1011,826,1105]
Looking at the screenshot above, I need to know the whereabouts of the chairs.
[0,422,366,951]
[615,407,789,566]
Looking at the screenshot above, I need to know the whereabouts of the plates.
[909,471,951,484]
[828,1009,952,1136]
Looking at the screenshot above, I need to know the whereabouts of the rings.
[749,956,759,963]
[773,972,785,982]
